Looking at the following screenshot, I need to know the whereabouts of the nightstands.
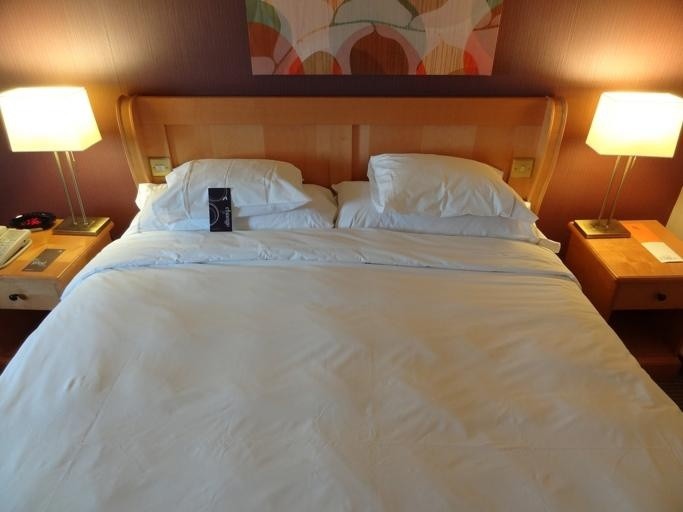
[570,219,682,379]
[0,216,116,367]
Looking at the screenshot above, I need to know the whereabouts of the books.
[22,248,66,273]
[207,186,232,233]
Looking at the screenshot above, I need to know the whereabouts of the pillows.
[151,158,313,223]
[135,181,338,228]
[330,182,562,253]
[366,153,540,222]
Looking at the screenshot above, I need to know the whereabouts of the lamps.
[575,90,683,239]
[0,86,111,236]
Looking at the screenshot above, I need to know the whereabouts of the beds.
[1,76,682,512]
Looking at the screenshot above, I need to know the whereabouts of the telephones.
[0,226,32,268]
